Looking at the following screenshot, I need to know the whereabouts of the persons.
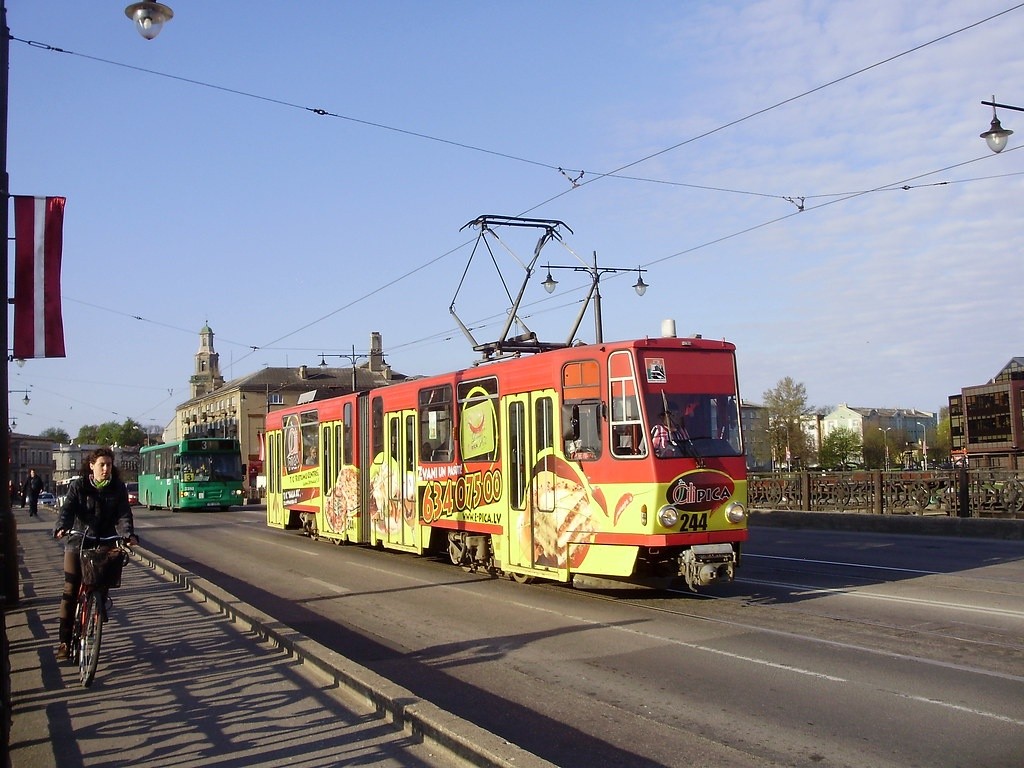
[53,448,140,662]
[9,479,15,508]
[17,481,26,508]
[20,468,44,517]
[184,463,192,472]
[649,400,689,449]
[306,446,318,466]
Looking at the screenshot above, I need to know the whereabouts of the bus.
[265,215,750,594]
[138,437,247,512]
[55,476,81,511]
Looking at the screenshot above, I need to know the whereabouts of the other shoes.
[57,642,70,659]
[101,606,109,622]
[29,512,38,517]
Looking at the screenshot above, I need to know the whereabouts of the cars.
[123,481,143,506]
[39,492,56,507]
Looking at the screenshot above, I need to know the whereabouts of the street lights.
[917,422,927,470]
[878,427,892,471]
[539,250,650,346]
[133,425,162,447]
[318,344,390,393]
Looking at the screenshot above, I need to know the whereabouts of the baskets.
[81,549,123,589]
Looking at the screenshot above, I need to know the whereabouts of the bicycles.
[59,524,134,689]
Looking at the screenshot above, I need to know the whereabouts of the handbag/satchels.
[82,547,129,589]
